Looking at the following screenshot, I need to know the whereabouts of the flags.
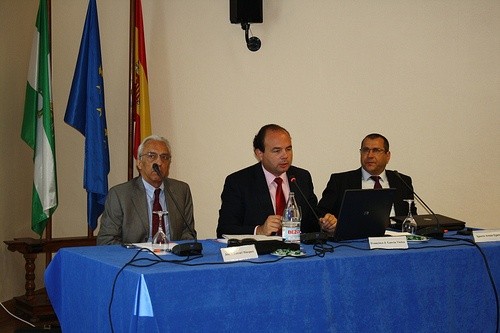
[130,0,154,160]
[19,0,58,238]
[64,0,110,232]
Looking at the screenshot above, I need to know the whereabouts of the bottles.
[281,192,300,245]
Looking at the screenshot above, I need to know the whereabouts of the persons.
[95,136,197,244]
[215,123,338,240]
[316,133,418,233]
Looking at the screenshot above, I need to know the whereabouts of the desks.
[44,227,500,333]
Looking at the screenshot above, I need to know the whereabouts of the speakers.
[229,0,263,24]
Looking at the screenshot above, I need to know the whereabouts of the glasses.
[139,152,170,160]
[359,148,387,154]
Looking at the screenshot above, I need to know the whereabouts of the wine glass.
[153,211,170,253]
[402,200,417,234]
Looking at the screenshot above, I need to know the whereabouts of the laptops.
[316,188,397,242]
[386,214,466,236]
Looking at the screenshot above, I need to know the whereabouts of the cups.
[285,206,302,222]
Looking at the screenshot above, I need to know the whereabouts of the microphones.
[289,174,329,245]
[393,169,444,238]
[152,163,203,255]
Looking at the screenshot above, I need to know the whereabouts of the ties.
[152,188,166,244]
[369,176,383,190]
[273,177,286,236]
